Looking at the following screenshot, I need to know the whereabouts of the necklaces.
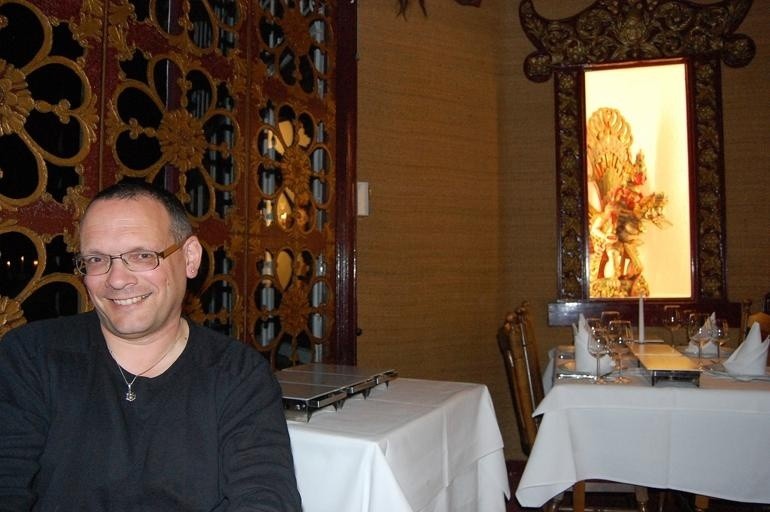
[100,316,183,402]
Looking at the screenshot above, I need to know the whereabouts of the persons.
[0,177,303,512]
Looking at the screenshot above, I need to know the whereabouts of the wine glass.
[587,329,609,385]
[687,313,714,373]
[661,305,684,349]
[682,310,699,340]
[584,318,602,338]
[706,319,729,366]
[599,311,621,358]
[607,321,633,385]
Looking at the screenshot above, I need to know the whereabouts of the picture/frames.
[516,0,757,328]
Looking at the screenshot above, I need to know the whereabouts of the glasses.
[72,233,195,276]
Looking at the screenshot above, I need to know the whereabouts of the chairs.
[740,292,770,349]
[495,300,671,512]
[694,494,710,512]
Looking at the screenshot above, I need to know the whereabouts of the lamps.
[357,181,369,216]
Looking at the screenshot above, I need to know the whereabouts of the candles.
[638,293,644,342]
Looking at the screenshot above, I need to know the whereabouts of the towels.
[577,312,593,341]
[688,312,718,354]
[572,323,613,376]
[723,335,769,376]
[724,322,762,363]
